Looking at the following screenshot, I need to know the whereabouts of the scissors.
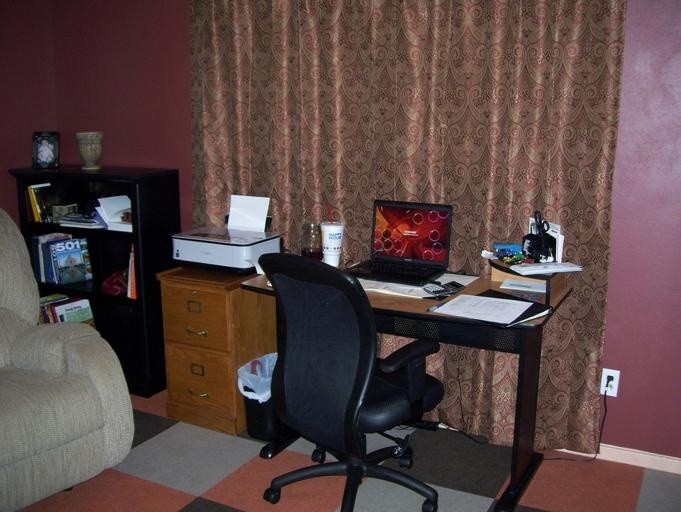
[535,211,549,236]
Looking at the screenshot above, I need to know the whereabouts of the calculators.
[425,281,464,296]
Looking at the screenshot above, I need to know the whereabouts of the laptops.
[340,199,453,287]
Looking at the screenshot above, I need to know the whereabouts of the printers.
[171,214,284,278]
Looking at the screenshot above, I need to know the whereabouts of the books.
[24,181,139,329]
[474,288,554,329]
[528,216,566,263]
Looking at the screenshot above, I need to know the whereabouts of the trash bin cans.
[238,352,292,440]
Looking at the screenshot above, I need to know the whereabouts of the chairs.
[257,252,446,512]
[0,207,136,511]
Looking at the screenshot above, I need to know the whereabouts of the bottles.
[301,223,322,254]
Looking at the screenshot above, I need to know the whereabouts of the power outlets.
[599,367,621,398]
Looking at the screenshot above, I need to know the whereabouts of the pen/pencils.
[427,301,448,313]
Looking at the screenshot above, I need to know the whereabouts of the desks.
[240,271,575,512]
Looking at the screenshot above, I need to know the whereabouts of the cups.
[320,223,345,268]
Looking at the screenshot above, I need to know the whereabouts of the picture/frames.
[31,130,61,170]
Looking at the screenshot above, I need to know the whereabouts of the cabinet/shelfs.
[154,266,280,437]
[7,164,181,399]
